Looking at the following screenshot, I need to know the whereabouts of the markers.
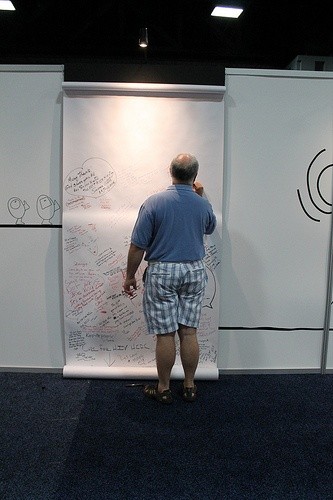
[124,383,144,388]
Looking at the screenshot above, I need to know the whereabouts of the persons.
[123,153,217,403]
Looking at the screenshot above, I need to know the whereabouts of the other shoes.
[182,384,199,403]
[142,384,174,404]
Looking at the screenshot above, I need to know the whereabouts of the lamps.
[139,28,149,47]
